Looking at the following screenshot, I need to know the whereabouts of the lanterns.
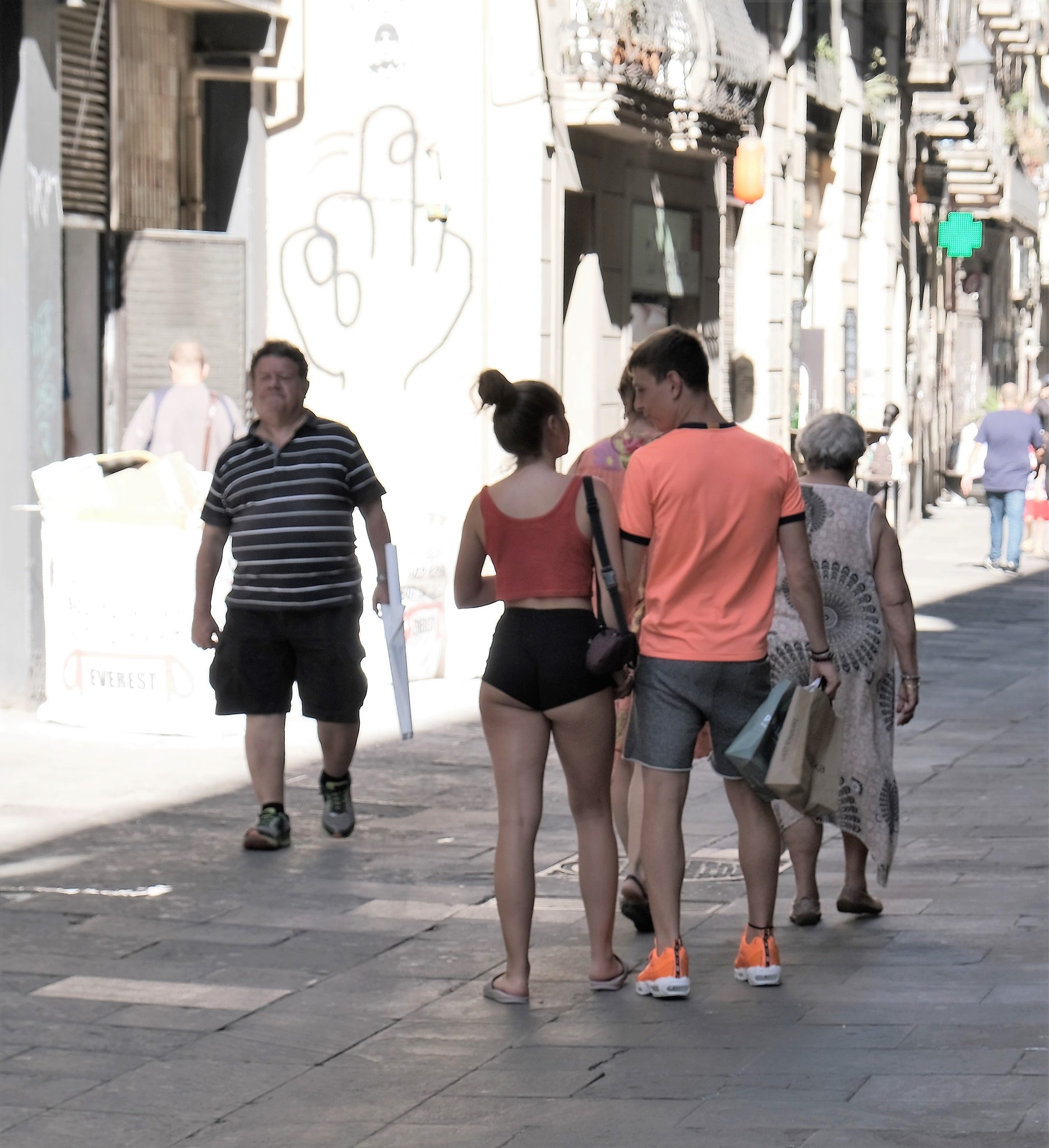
[732,135,766,206]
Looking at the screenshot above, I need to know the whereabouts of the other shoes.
[836,886,883,915]
[974,557,1002,568]
[1001,561,1020,575]
[790,895,821,926]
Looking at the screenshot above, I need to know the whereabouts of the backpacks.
[867,430,897,487]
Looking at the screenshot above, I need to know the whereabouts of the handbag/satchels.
[586,628,638,679]
[764,676,836,810]
[725,676,793,804]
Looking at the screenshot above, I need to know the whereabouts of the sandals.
[620,874,653,934]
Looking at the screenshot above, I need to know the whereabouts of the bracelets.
[810,653,834,662]
[901,682,920,688]
[900,674,921,681]
[376,574,387,585]
[811,647,829,655]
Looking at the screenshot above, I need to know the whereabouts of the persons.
[120,339,248,476]
[961,373,1049,571]
[566,324,919,999]
[190,340,391,850]
[453,370,638,1005]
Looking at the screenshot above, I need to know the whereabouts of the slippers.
[484,970,530,1004]
[590,954,630,990]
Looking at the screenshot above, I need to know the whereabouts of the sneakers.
[734,922,782,987]
[242,802,290,850]
[320,772,355,838]
[636,937,691,997]
